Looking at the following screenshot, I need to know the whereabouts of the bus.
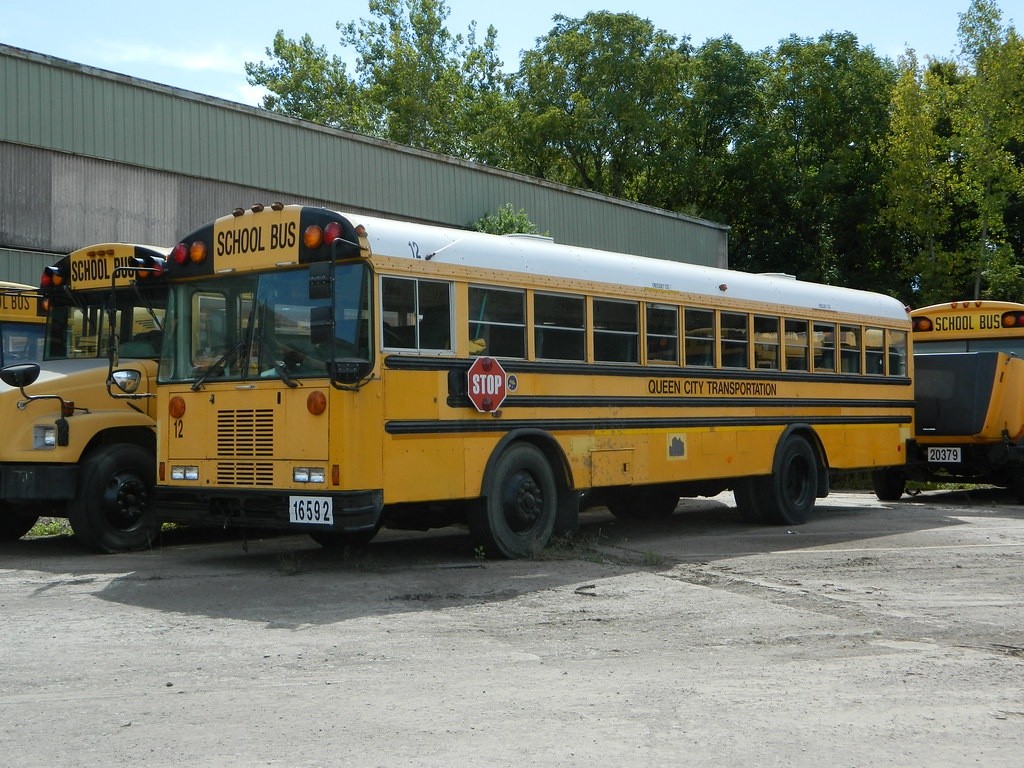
[903,299,1024,504]
[0,246,188,550]
[154,203,915,560]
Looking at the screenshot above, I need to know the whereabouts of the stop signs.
[467,357,507,416]
